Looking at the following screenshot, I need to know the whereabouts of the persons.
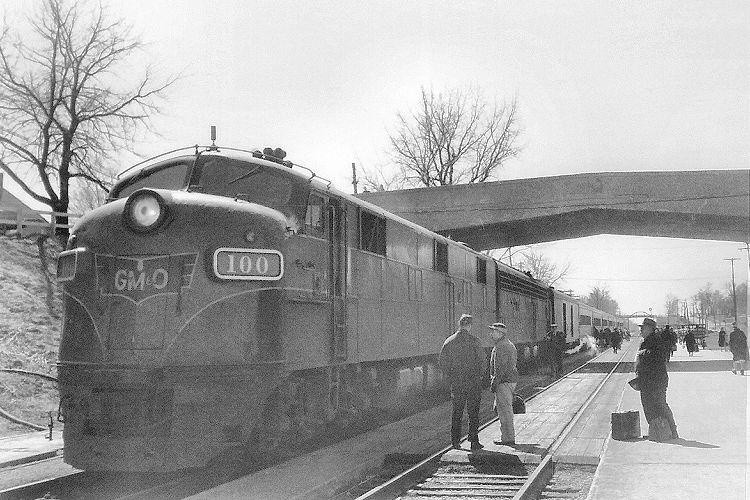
[488,321,519,447]
[437,313,488,451]
[634,318,678,441]
[669,326,677,357]
[661,323,672,362]
[729,322,750,376]
[683,330,696,357]
[716,327,729,353]
[536,325,630,379]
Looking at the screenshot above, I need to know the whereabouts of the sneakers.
[469,440,486,451]
[492,437,518,447]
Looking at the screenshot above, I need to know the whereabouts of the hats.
[487,321,509,335]
[637,316,659,333]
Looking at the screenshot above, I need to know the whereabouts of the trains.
[55,126,625,475]
